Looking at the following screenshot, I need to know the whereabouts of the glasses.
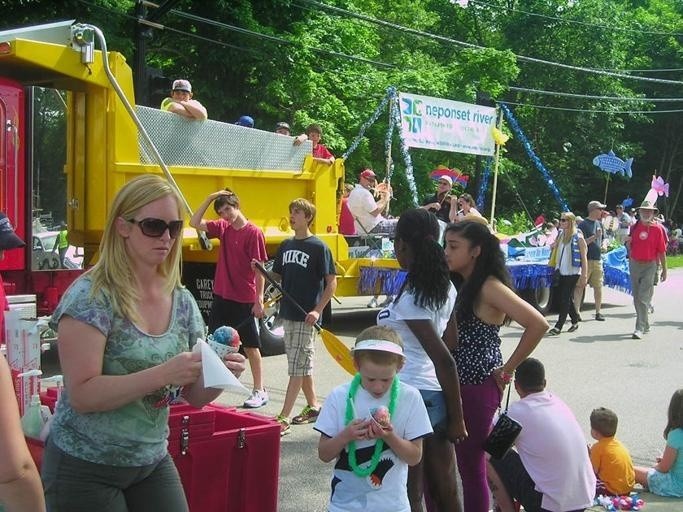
[559,219,568,222]
[129,217,184,240]
[365,177,375,182]
[439,181,447,186]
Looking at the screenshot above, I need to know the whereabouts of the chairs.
[353,213,391,259]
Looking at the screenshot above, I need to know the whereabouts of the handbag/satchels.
[484,382,522,460]
[551,269,561,287]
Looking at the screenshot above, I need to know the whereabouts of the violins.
[439,192,463,206]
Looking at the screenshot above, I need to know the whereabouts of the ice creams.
[210,325,241,347]
[372,405,390,424]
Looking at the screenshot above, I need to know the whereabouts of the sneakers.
[595,313,604,321]
[292,404,321,425]
[277,416,291,436]
[244,388,268,408]
[632,330,642,339]
[549,325,579,336]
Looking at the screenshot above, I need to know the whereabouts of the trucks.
[0,20,627,356]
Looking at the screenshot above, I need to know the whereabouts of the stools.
[343,233,361,250]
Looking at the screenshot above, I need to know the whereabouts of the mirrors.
[27,83,86,270]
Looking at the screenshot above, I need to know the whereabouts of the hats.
[439,175,452,186]
[274,121,290,131]
[172,79,192,92]
[350,340,406,361]
[235,116,254,128]
[361,169,376,178]
[635,201,659,217]
[0,211,25,250]
[586,201,607,210]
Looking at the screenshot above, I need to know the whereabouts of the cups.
[366,409,389,432]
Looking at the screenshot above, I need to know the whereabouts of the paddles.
[255,262,359,377]
